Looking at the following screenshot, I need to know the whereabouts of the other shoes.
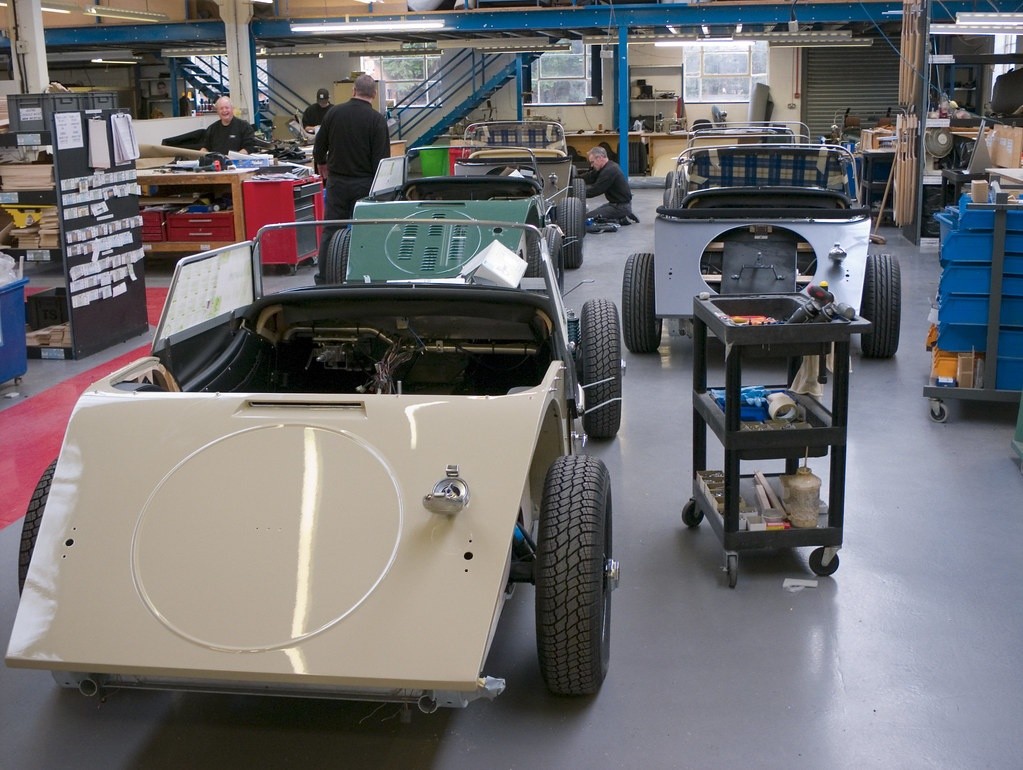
[628,212,640,223]
[619,216,633,225]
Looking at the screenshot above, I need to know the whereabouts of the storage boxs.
[860,129,893,151]
[6,91,119,132]
[27,287,69,331]
[932,191,1023,391]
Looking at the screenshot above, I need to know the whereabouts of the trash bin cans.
[419,144,448,177]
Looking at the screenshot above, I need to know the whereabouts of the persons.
[573,147,640,226]
[312,75,390,287]
[200,95,254,155]
[302,90,337,135]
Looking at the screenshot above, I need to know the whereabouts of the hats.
[316,89,329,104]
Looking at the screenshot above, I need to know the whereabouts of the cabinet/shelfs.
[136,167,259,253]
[449,126,778,177]
[860,152,895,221]
[140,77,185,118]
[241,175,325,277]
[682,291,873,590]
[0,107,150,360]
[628,64,684,118]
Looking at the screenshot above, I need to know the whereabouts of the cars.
[453,119,587,270]
[4,217,618,714]
[325,174,565,299]
[621,119,902,364]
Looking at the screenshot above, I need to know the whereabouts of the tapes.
[768,396,798,422]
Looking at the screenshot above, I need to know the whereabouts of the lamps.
[82,0,169,22]
[788,0,799,33]
[288,19,445,32]
[0,0,79,14]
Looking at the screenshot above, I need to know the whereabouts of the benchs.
[454,122,573,203]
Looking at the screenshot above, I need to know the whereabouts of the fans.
[923,127,954,176]
